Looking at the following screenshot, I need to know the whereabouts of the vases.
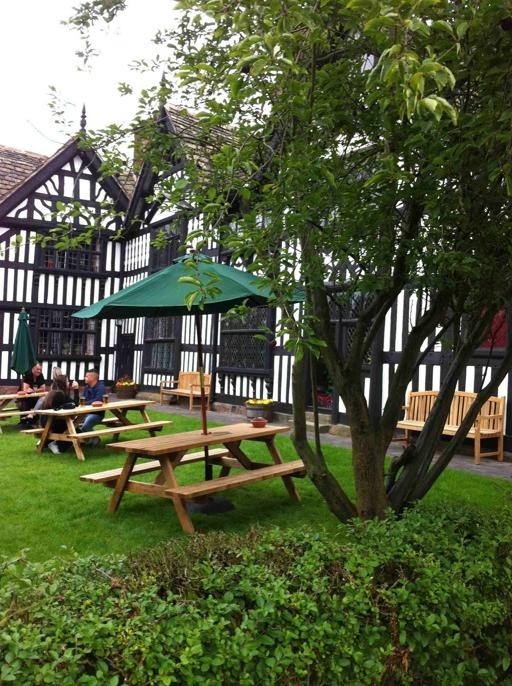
[116,386,137,399]
[247,404,273,423]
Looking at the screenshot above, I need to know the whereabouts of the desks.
[0,391,49,433]
[34,399,156,461]
[106,423,302,533]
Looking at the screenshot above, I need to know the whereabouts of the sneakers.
[48,441,61,455]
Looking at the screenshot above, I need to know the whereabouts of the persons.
[16,362,106,455]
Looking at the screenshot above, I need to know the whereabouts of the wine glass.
[33,385,38,394]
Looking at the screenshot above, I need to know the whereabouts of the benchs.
[0,407,19,411]
[0,410,40,417]
[160,371,212,412]
[80,447,230,483]
[390,391,505,465]
[20,417,119,435]
[165,459,306,499]
[66,420,173,439]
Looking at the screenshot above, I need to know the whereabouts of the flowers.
[245,398,273,406]
[115,377,136,388]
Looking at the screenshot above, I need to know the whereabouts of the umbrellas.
[70,250,306,482]
[9,307,38,375]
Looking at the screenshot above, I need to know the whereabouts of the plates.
[91,403,103,407]
[18,392,26,395]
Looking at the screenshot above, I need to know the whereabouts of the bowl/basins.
[250,419,268,428]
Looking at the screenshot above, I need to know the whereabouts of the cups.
[103,395,109,404]
[80,398,86,407]
